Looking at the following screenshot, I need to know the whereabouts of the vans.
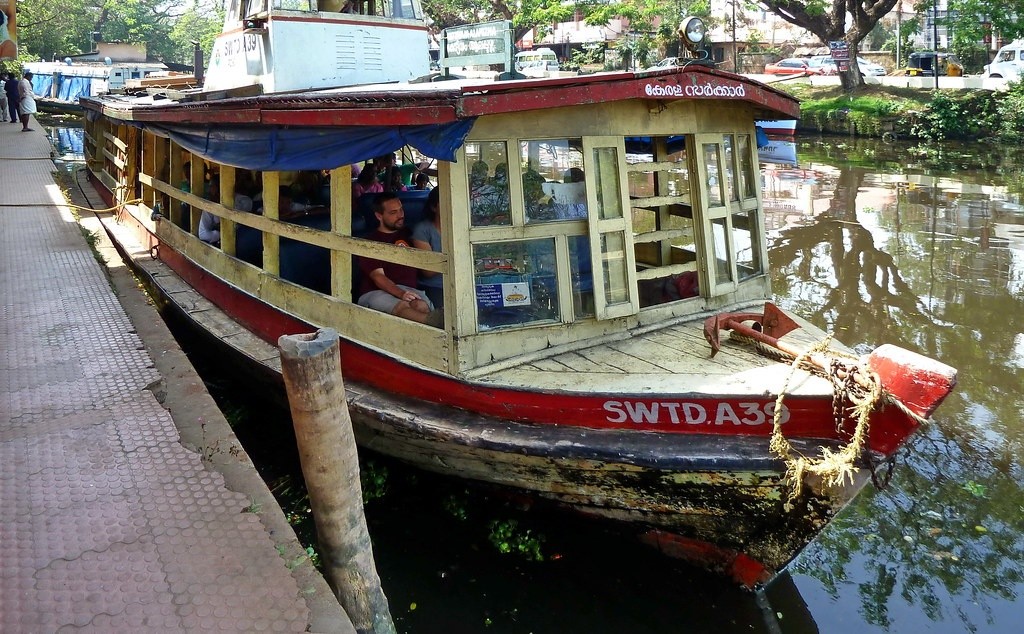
[980,39,1024,77]
[905,52,965,76]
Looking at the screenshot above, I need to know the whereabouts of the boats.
[74,0,959,590]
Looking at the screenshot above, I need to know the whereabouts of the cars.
[765,54,888,77]
[521,59,561,72]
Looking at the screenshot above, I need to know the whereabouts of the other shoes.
[22,128,36,132]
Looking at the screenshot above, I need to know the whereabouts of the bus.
[515,47,558,70]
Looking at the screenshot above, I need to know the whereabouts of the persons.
[151,153,585,329]
[17,72,37,131]
[4,73,23,123]
[0,73,8,122]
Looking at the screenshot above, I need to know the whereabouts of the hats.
[414,174,429,182]
